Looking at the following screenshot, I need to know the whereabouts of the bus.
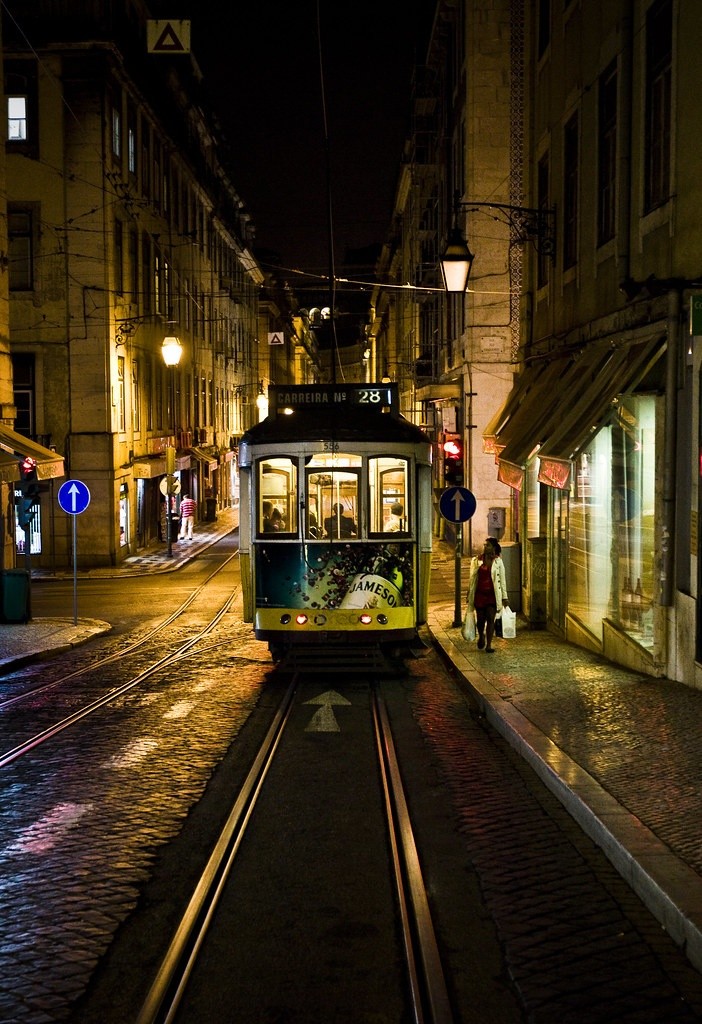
[233,380,433,666]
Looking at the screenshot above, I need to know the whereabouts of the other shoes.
[477,634,485,649]
[485,648,495,653]
[180,537,184,540]
[189,538,192,540]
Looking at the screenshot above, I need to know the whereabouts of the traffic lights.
[166,474,179,495]
[20,458,40,504]
[435,488,448,518]
[443,438,465,487]
[20,496,38,529]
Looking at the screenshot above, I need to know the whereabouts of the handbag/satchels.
[494,616,502,637]
[501,606,517,639]
[461,610,476,641]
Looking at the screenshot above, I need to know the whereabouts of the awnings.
[0,424,65,484]
[189,447,218,471]
[482,332,668,491]
[0,449,22,484]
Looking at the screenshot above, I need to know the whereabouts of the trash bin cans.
[206,499,218,522]
[0,568,31,625]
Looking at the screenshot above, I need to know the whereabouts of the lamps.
[115,306,185,367]
[363,347,371,359]
[382,358,411,383]
[438,189,558,294]
[234,380,268,409]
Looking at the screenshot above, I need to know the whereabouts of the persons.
[179,494,197,540]
[262,501,281,533]
[466,538,508,653]
[325,502,357,539]
[383,503,405,532]
